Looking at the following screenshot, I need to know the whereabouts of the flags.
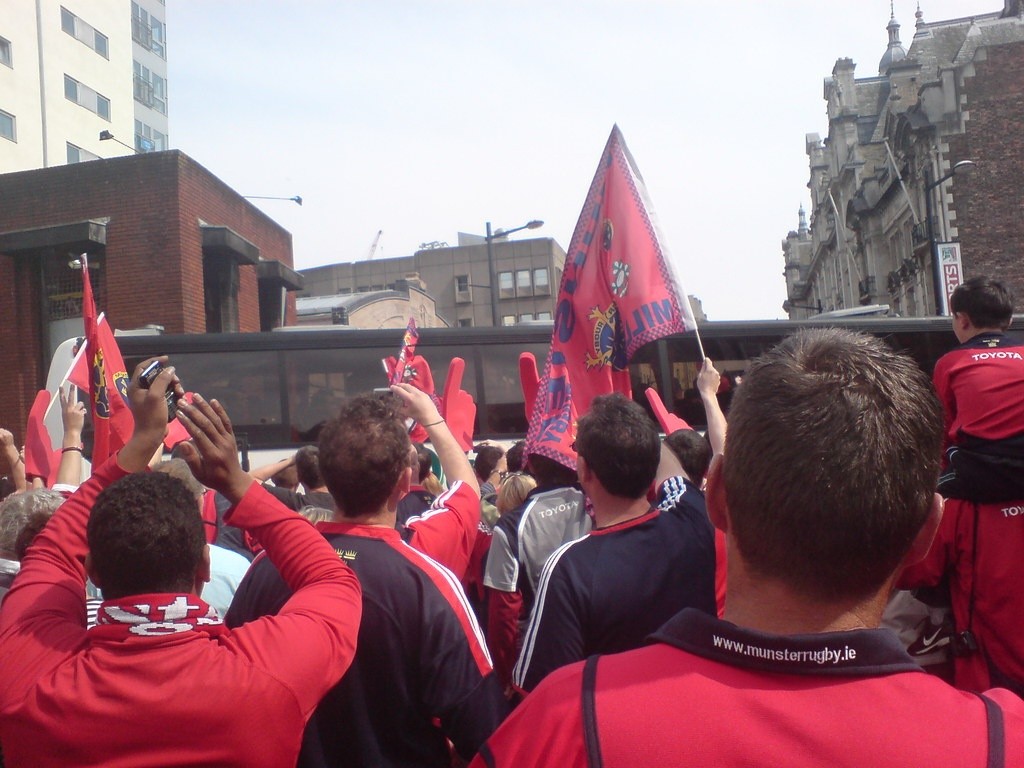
[67,126,697,480]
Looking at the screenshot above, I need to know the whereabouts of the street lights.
[486,219,547,326]
[921,159,980,316]
[781,299,822,316]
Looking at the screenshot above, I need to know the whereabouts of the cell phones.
[374,388,395,398]
[137,361,180,423]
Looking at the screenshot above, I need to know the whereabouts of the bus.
[42,313,1024,499]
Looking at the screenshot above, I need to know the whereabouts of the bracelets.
[423,418,446,428]
[62,446,83,452]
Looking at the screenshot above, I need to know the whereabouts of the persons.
[219,396,506,768]
[469,326,1023,768]
[0,355,363,768]
[0,274,1024,699]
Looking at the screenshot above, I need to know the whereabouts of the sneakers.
[906,615,950,657]
